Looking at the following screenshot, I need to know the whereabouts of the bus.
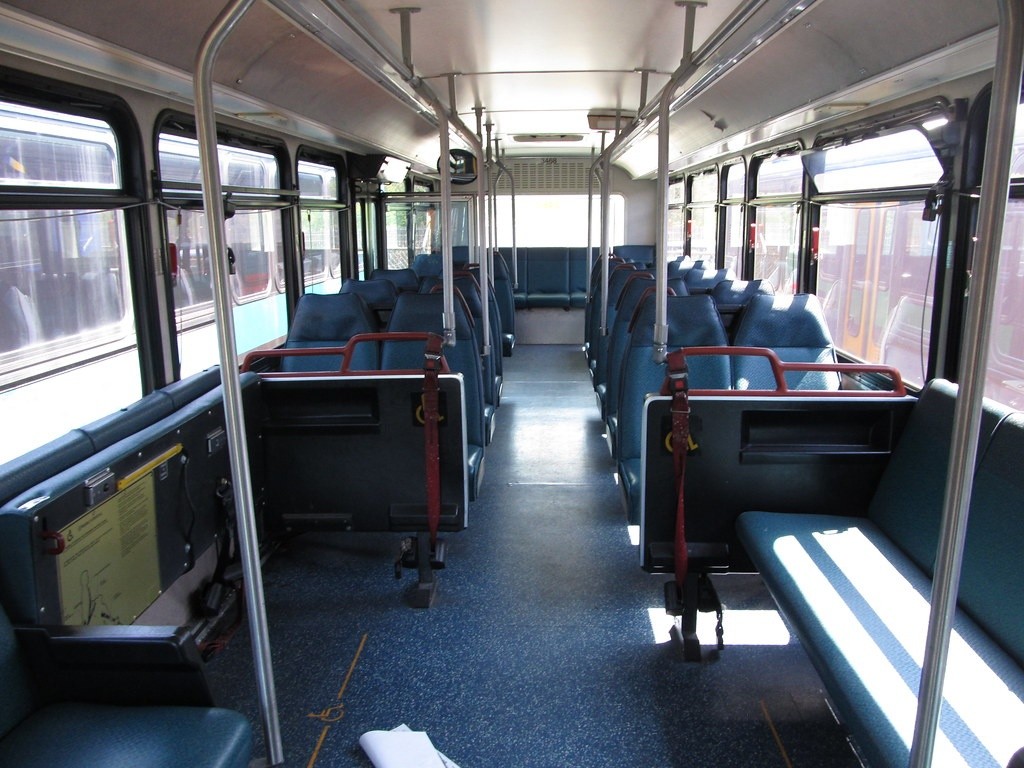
[1,105,344,467]
[665,134,1024,419]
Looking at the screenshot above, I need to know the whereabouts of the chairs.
[274,239,844,528]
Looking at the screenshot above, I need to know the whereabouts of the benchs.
[0,375,1023,768]
